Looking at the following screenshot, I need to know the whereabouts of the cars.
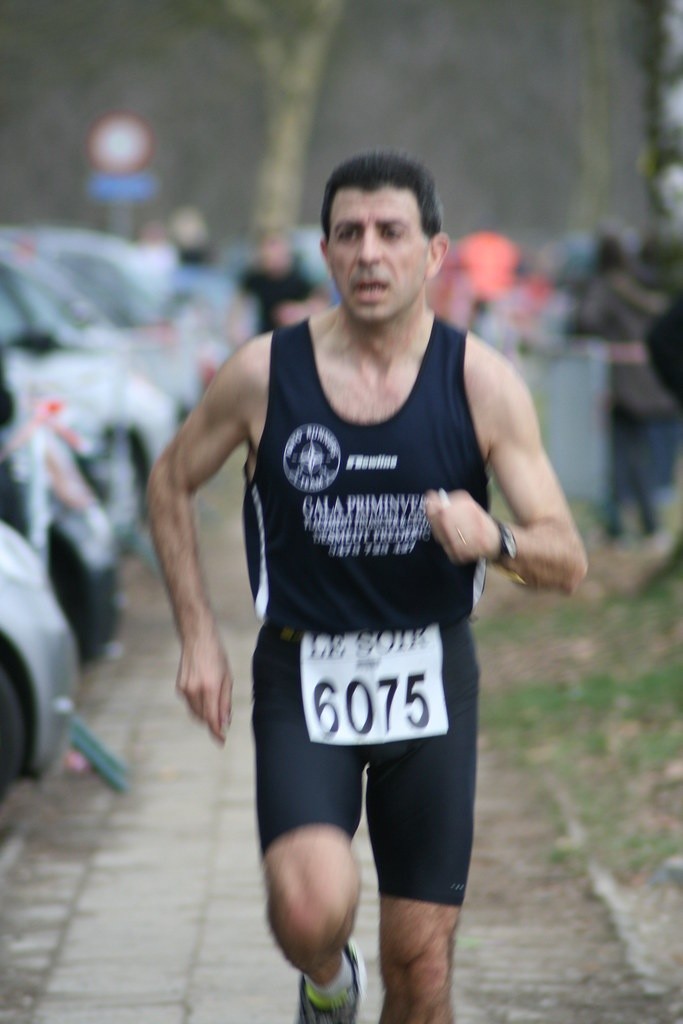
[0,231,258,800]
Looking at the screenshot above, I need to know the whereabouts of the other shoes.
[295,938,366,1024]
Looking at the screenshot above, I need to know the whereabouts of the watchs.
[491,515,520,565]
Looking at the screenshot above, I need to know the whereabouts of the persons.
[564,148,683,544]
[148,153,591,1024]
[228,228,332,336]
